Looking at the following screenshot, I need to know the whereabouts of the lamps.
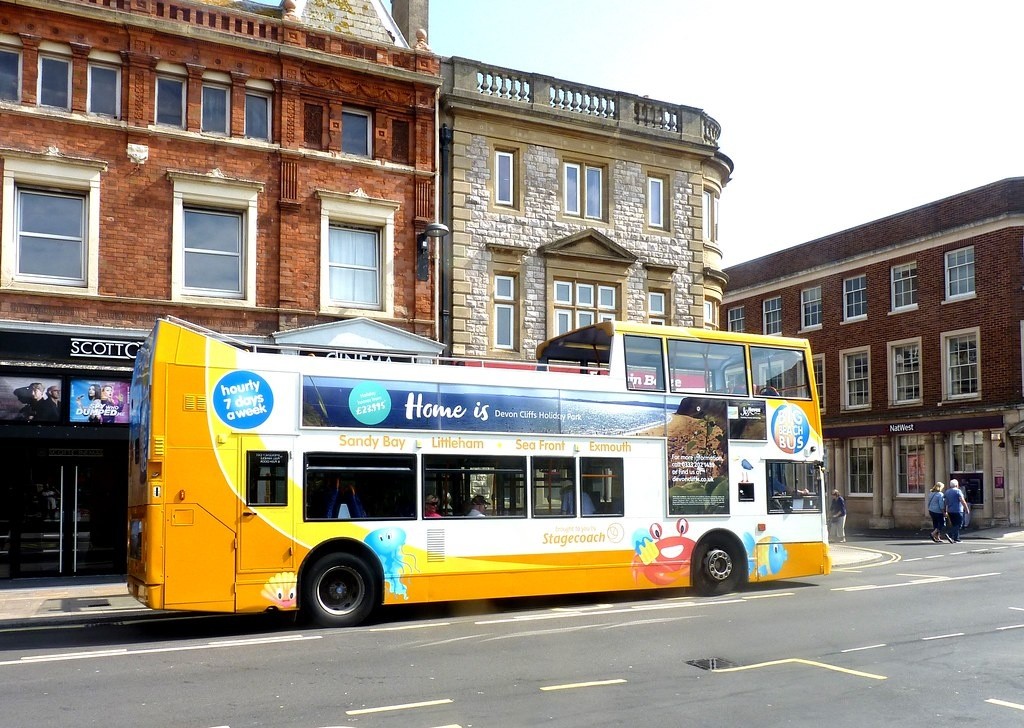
[416,223,450,281]
[990,434,1006,449]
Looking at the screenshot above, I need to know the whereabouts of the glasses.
[426,501,439,506]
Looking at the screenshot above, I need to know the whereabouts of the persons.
[423,481,598,519]
[768,472,970,544]
[15,383,123,423]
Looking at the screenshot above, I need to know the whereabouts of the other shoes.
[929,533,936,542]
[838,539,846,543]
[954,540,963,544]
[935,538,943,542]
[945,534,954,544]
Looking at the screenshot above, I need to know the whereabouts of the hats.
[559,480,574,495]
[471,495,491,506]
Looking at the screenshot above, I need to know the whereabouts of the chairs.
[323,478,339,518]
[760,386,780,396]
[344,483,368,518]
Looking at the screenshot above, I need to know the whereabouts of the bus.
[125,314,831,631]
